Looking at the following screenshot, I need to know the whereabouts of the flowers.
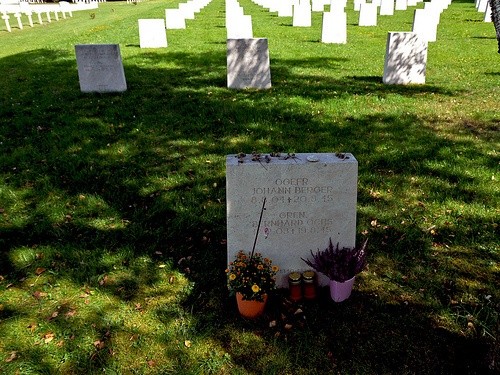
[299,235,369,283]
[224,249,281,304]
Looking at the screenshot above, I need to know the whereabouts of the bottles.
[288,272,302,303]
[301,270,317,300]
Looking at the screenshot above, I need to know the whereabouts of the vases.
[236,292,268,320]
[329,275,356,303]
